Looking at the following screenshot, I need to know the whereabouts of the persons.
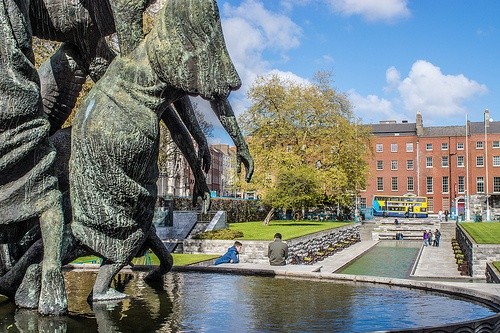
[438,209,443,222]
[435,229,441,247]
[214,241,242,265]
[267,233,289,265]
[423,230,428,247]
[385,211,389,217]
[0,0,110,316]
[445,208,449,221]
[428,229,433,245]
[394,219,398,225]
[433,232,437,246]
[383,210,385,217]
[0,0,255,302]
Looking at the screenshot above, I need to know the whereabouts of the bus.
[370,194,428,218]
